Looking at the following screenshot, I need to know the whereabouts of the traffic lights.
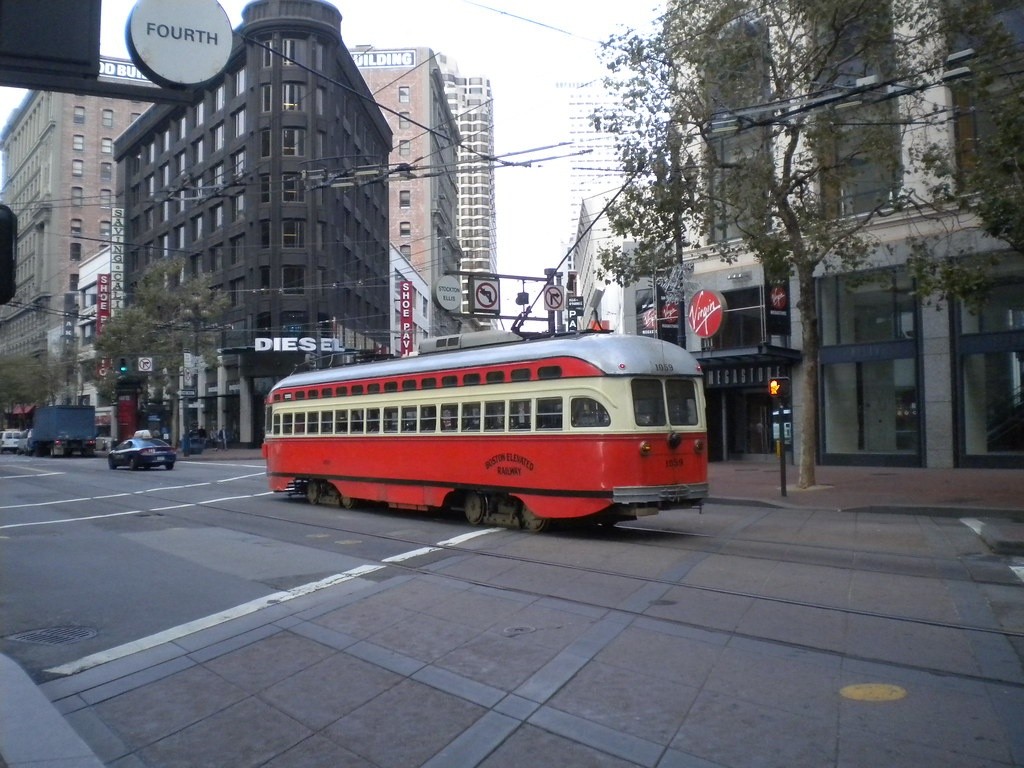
[119,357,128,375]
[768,377,791,399]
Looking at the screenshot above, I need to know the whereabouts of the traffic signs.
[177,389,197,398]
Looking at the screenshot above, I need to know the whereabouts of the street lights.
[180,293,210,456]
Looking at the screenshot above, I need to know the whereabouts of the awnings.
[12,403,35,415]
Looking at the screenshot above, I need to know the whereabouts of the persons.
[153,427,160,438]
[198,425,229,452]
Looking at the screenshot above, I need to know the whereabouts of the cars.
[106,429,177,472]
[0,428,32,455]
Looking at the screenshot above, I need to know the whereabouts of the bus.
[262,158,709,534]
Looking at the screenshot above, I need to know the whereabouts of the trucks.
[27,404,98,458]
